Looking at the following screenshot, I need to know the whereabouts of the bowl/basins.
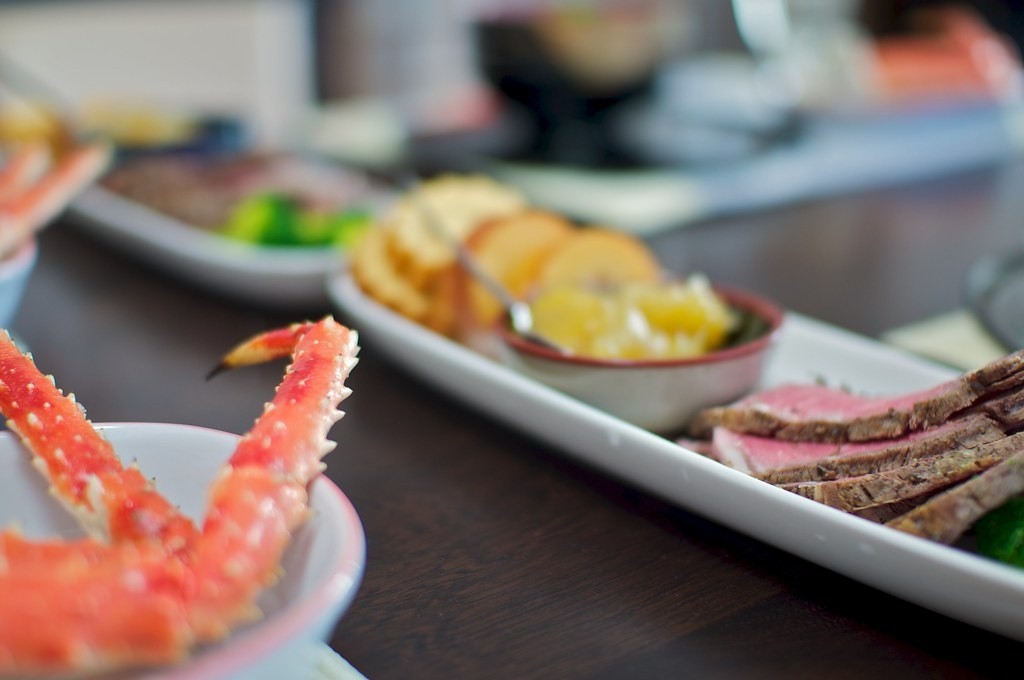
[494,284,782,434]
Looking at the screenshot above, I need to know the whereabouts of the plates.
[326,266,1022,632]
[68,185,329,304]
[5,423,365,678]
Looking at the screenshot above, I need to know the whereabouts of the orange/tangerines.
[462,214,653,317]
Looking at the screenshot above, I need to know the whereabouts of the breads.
[348,174,523,324]
